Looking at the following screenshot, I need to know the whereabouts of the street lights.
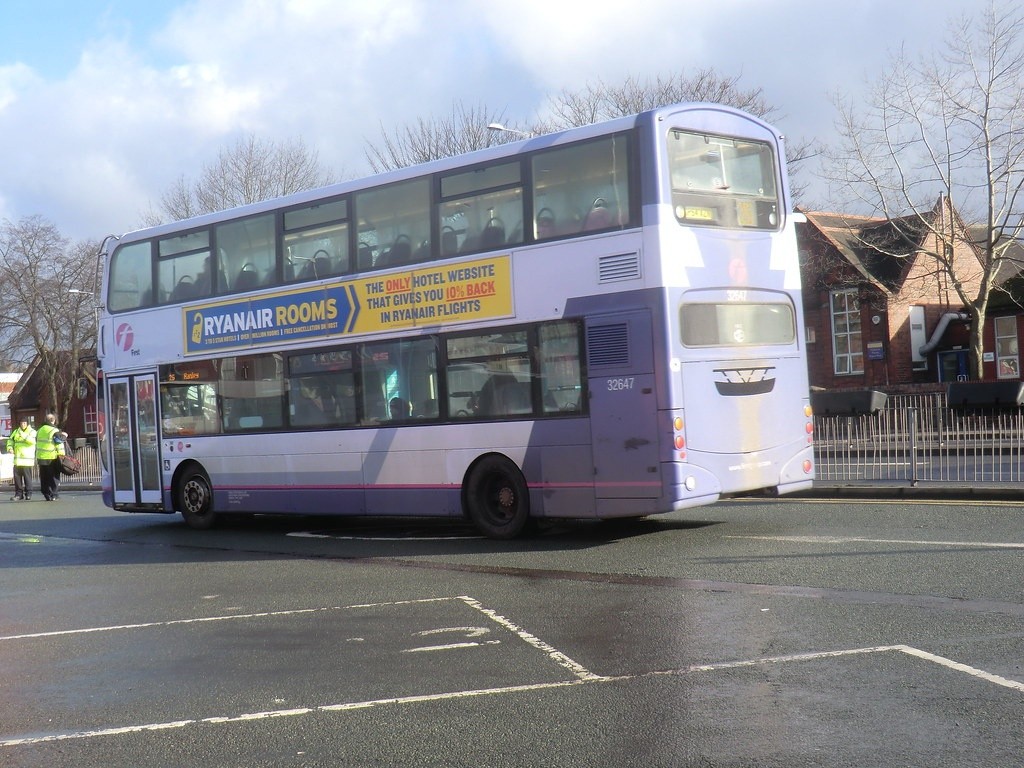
[69,290,98,330]
[487,122,533,141]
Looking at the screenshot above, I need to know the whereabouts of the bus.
[94,102,817,538]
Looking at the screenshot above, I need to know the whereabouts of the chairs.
[140,197,613,309]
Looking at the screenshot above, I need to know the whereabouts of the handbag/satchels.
[57,455,80,476]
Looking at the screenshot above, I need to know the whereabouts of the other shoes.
[25,496,31,500]
[45,497,59,501]
[10,495,24,501]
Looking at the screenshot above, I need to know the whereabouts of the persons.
[191,256,229,297]
[389,397,410,420]
[6,417,37,501]
[36,414,68,501]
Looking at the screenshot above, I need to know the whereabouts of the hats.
[19,417,28,422]
